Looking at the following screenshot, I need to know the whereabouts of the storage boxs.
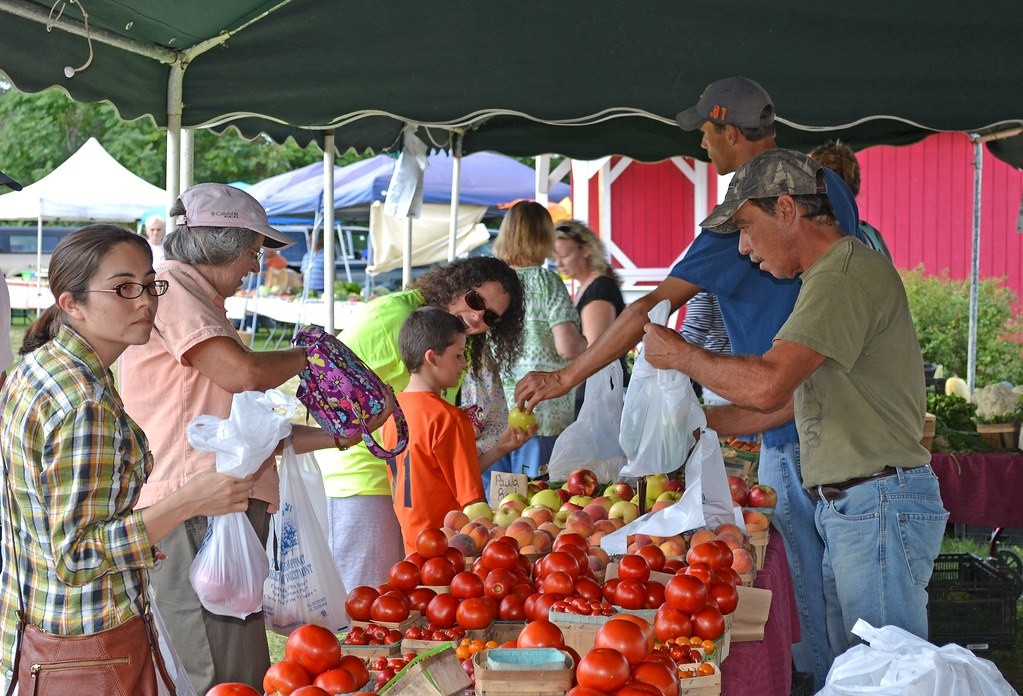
[238,331,252,347]
[926,552,1018,650]
[487,648,566,670]
[549,605,622,624]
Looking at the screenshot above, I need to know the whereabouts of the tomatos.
[190,549,268,614]
[204,614,717,696]
[346,528,742,643]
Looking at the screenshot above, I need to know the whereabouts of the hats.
[677,77,777,131]
[174,184,294,248]
[699,146,827,227]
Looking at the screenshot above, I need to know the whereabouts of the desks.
[929,451,1023,556]
[718,533,802,696]
[4,276,364,350]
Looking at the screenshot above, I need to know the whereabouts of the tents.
[228,149,571,349]
[0,136,174,286]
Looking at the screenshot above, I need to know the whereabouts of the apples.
[461,466,778,524]
[508,406,537,435]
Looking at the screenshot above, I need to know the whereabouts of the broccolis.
[976,382,1023,422]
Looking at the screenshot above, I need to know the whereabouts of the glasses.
[247,247,265,264]
[462,286,503,329]
[86,278,169,299]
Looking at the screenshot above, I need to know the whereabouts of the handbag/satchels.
[185,391,292,618]
[263,437,355,639]
[8,605,180,695]
[816,618,1018,696]
[291,324,409,459]
[547,359,625,487]
[614,296,706,480]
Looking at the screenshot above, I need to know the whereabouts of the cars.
[0,226,502,293]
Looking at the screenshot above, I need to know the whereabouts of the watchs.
[334,437,350,451]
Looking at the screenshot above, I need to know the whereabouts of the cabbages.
[258,285,283,296]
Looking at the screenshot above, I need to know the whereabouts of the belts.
[809,464,900,505]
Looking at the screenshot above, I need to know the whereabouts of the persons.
[0,172,253,696]
[301,255,541,598]
[114,184,393,696]
[513,77,950,696]
[492,201,629,481]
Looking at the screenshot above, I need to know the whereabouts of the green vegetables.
[925,390,992,454]
[333,280,363,301]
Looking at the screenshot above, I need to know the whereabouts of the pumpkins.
[945,374,970,403]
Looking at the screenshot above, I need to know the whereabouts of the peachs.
[438,504,769,575]
